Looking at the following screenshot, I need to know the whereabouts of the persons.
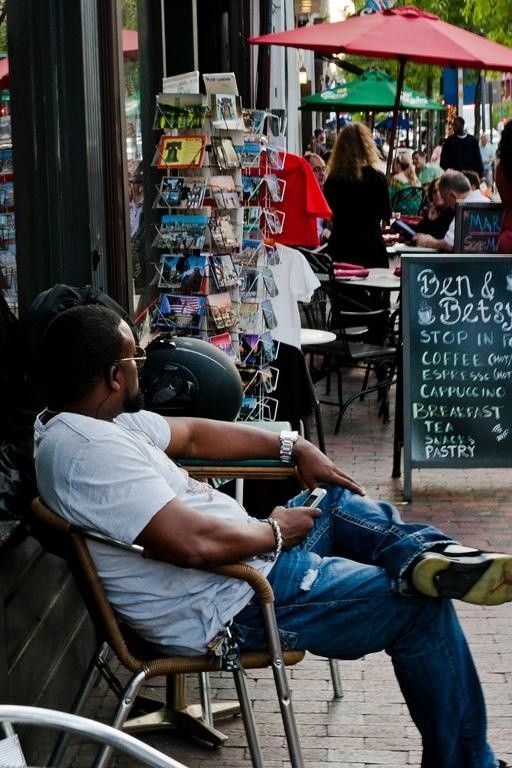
[323,121,392,368]
[30,303,511,768]
[303,111,511,255]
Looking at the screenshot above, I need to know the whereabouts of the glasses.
[115,346,147,368]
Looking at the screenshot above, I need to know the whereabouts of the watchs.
[277,428,300,465]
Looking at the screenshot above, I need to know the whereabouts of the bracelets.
[255,518,284,562]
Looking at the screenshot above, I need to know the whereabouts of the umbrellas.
[298,66,448,136]
[247,0,512,179]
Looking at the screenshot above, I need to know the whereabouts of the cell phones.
[302,487,328,507]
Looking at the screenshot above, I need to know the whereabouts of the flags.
[164,294,201,316]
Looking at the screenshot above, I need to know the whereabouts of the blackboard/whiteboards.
[401,254,512,468]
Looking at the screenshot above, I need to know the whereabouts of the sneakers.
[411,543,511,605]
